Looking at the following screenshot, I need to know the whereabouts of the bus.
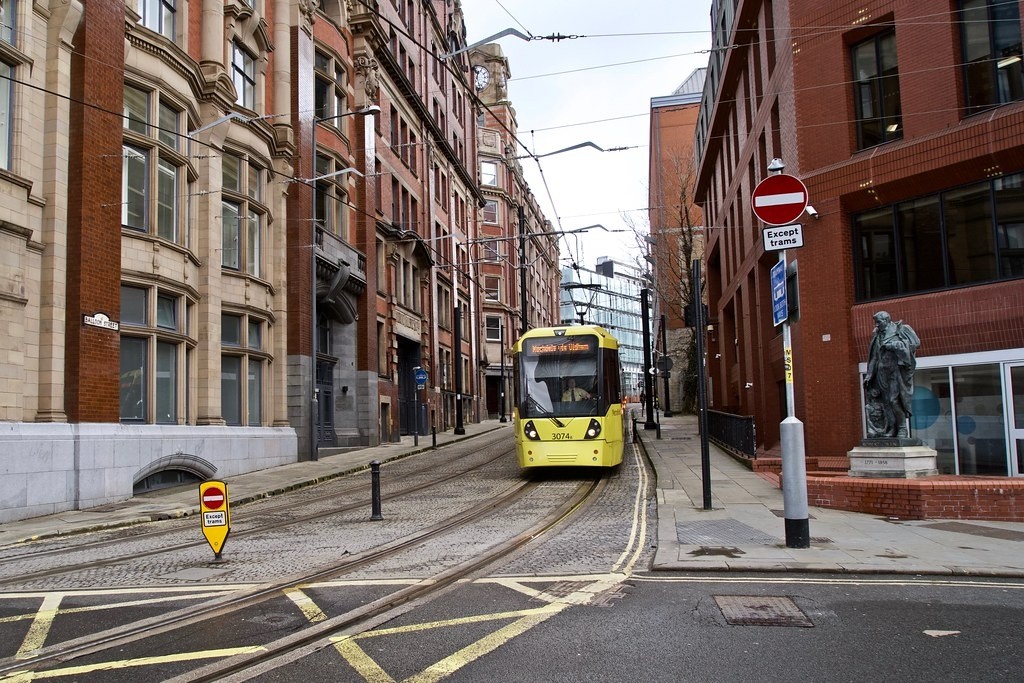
[511,324,627,469]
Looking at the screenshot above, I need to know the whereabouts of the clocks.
[473,65,490,91]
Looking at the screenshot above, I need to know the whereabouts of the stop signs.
[202,488,225,510]
[750,174,809,227]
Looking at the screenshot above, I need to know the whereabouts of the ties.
[571,390,575,402]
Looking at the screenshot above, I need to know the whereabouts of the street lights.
[308,105,383,459]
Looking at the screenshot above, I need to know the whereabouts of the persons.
[640,391,646,410]
[561,379,591,402]
[865,311,920,437]
